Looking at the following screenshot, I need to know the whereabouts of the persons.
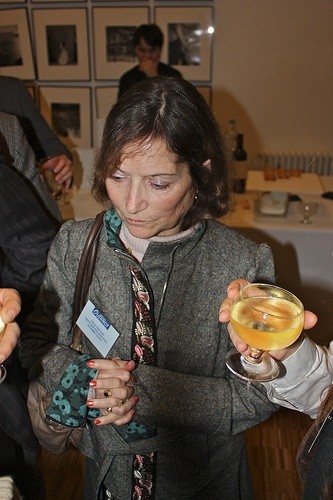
[0,24,192,500]
[219,279,333,500]
[22,76,274,500]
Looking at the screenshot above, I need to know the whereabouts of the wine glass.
[225,283,304,383]
[298,201,318,225]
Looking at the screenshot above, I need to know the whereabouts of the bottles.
[224,120,239,165]
[232,133,248,194]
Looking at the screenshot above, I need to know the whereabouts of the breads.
[264,168,302,181]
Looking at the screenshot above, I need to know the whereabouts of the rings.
[104,389,112,399]
[106,408,113,413]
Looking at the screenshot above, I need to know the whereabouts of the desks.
[51,170,333,346]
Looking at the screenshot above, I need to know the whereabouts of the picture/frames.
[0,0,214,148]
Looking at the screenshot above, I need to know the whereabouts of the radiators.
[258,151,333,176]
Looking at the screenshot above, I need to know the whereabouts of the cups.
[38,154,75,207]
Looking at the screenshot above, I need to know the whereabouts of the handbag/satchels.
[26,333,85,456]
